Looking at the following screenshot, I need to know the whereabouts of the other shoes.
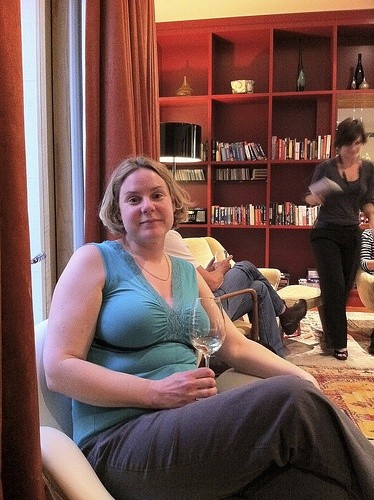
[334,347,350,362]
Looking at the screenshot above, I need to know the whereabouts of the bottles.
[176,75,193,96]
[351,53,371,90]
[296,50,305,91]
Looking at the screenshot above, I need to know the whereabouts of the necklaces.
[124,237,170,281]
[339,153,361,196]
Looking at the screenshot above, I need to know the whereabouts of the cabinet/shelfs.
[155,18,374,291]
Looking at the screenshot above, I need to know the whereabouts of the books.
[269,200,321,225]
[211,203,267,225]
[272,134,331,160]
[212,139,267,161]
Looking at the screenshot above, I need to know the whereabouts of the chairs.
[182,237,323,309]
[34,319,264,500]
[217,289,258,341]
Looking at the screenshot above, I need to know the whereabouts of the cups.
[215,249,228,264]
[231,80,255,94]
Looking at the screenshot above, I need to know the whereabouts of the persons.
[303,118,374,360]
[42,156,374,500]
[163,229,307,359]
[360,214,374,355]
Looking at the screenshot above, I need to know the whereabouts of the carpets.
[276,308,374,446]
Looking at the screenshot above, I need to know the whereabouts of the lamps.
[159,121,201,178]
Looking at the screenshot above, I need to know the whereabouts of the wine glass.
[189,297,227,401]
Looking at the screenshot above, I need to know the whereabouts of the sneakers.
[280,299,308,336]
